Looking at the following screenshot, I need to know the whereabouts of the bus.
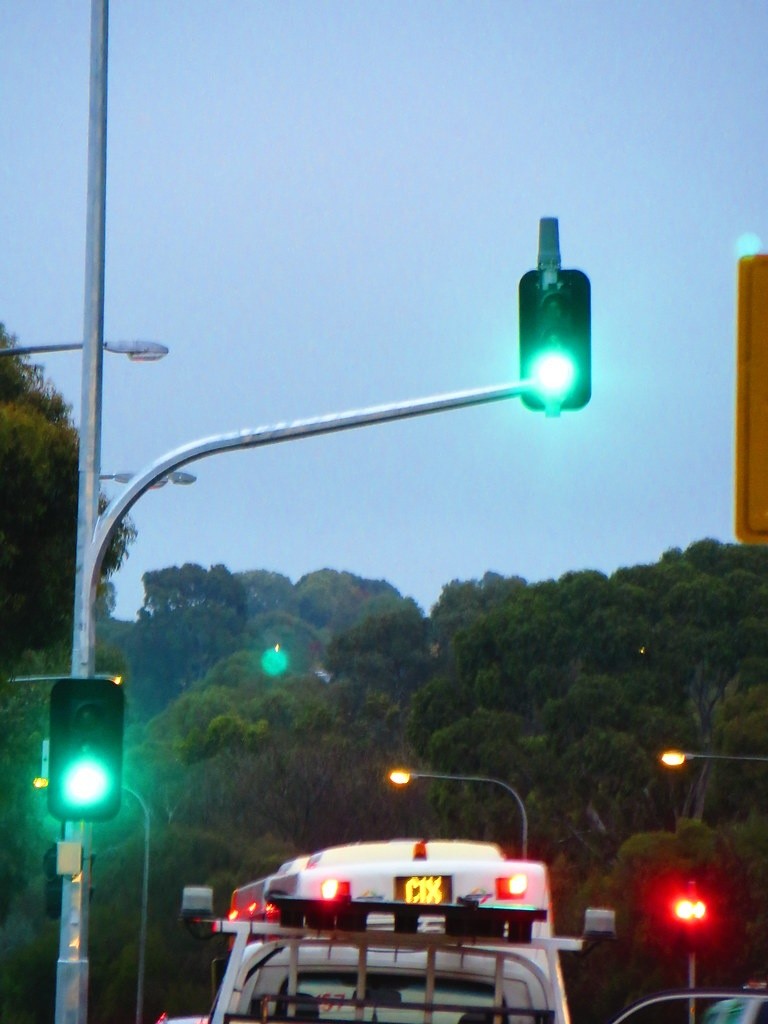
[227,840,554,952]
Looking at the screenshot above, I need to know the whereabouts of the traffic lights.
[47,678,124,821]
[519,269,591,412]
[674,899,707,920]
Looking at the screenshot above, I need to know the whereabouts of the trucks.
[179,885,616,1024]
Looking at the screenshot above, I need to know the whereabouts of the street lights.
[391,769,528,861]
[34,777,150,1024]
